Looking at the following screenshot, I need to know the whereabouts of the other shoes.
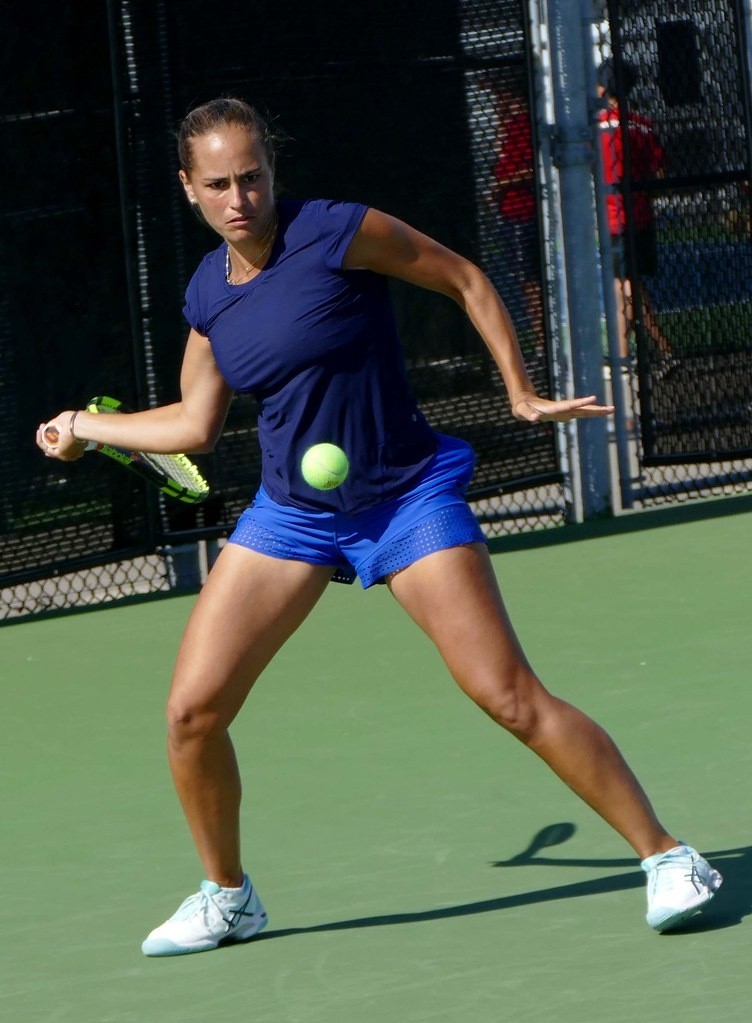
[656,353,680,379]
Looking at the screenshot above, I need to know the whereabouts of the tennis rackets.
[41,395,212,505]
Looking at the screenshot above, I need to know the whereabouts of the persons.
[33,96,724,957]
[499,54,552,372]
[597,57,685,383]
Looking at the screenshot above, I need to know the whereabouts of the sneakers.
[141,873,269,956]
[640,840,723,930]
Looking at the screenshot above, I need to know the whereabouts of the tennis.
[300,443,349,491]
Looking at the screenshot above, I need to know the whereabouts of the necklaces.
[225,220,279,286]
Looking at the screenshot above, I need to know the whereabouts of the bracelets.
[69,410,88,443]
